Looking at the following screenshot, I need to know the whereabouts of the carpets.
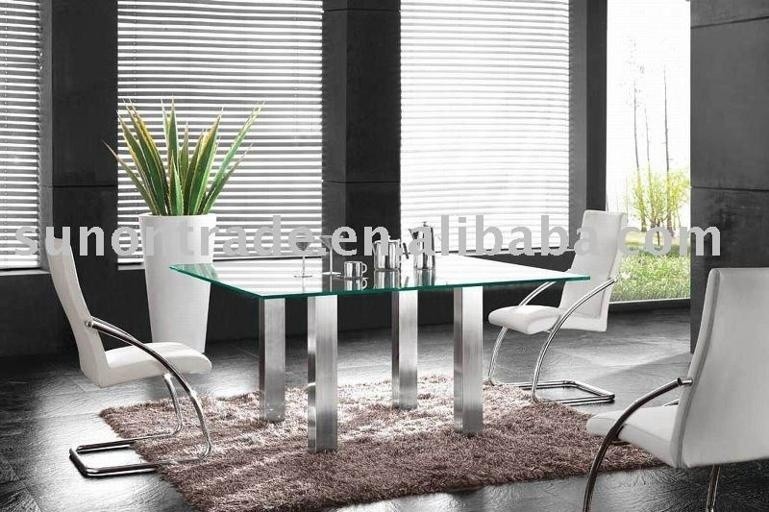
[98,372,670,512]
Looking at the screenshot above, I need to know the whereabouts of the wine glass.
[294,228,314,279]
[314,234,343,276]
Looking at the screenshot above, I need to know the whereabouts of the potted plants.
[90,80,267,357]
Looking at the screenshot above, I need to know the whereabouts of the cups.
[344,261,367,278]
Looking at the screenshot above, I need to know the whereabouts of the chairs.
[44,235,215,481]
[573,264,769,512]
[481,210,630,414]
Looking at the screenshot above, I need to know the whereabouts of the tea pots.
[369,237,409,270]
[409,220,435,270]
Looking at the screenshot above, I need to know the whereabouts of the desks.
[164,251,590,452]
